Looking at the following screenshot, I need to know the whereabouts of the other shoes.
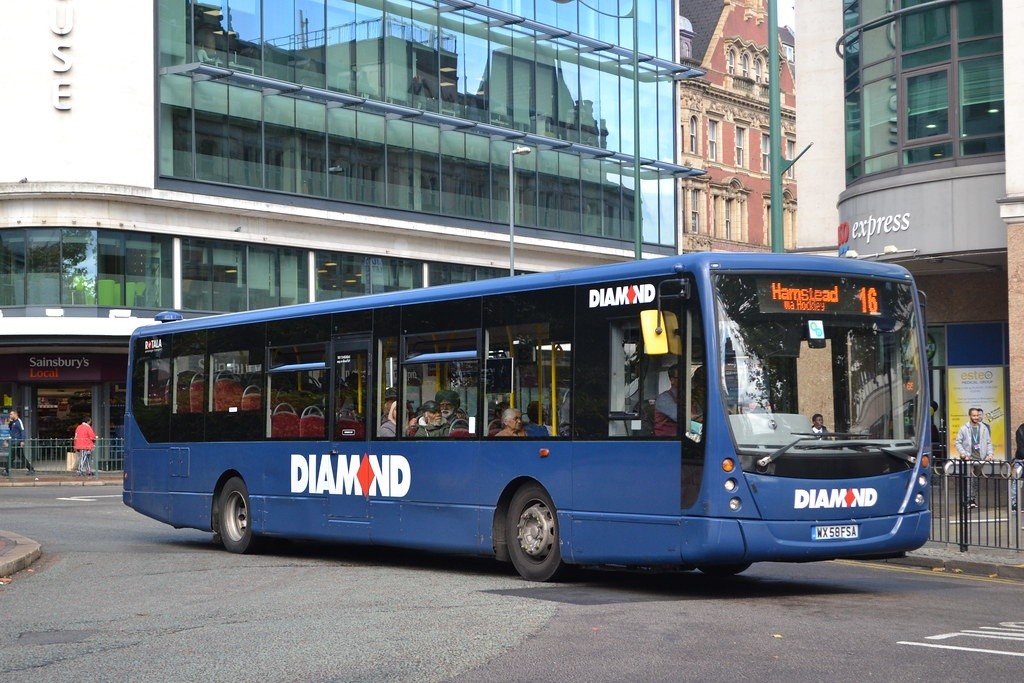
[2,471,9,477]
[26,469,36,476]
[1011,506,1018,512]
[968,500,978,509]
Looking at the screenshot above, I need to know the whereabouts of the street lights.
[509,145,532,277]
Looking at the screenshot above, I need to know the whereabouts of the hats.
[421,400,441,411]
[435,390,460,408]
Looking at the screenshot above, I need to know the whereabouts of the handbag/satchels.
[66,451,80,471]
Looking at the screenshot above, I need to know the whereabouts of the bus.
[121,249,936,584]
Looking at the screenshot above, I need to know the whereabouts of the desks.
[201,58,254,75]
[441,108,457,116]
[491,120,509,127]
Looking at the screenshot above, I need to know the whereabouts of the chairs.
[147,369,549,438]
[633,399,657,436]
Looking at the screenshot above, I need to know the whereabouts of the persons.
[746,399,765,413]
[977,408,992,437]
[1011,423,1024,513]
[955,407,993,509]
[653,363,704,437]
[341,384,570,437]
[73,416,96,476]
[812,413,832,440]
[0,409,36,476]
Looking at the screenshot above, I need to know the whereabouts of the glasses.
[440,403,452,407]
